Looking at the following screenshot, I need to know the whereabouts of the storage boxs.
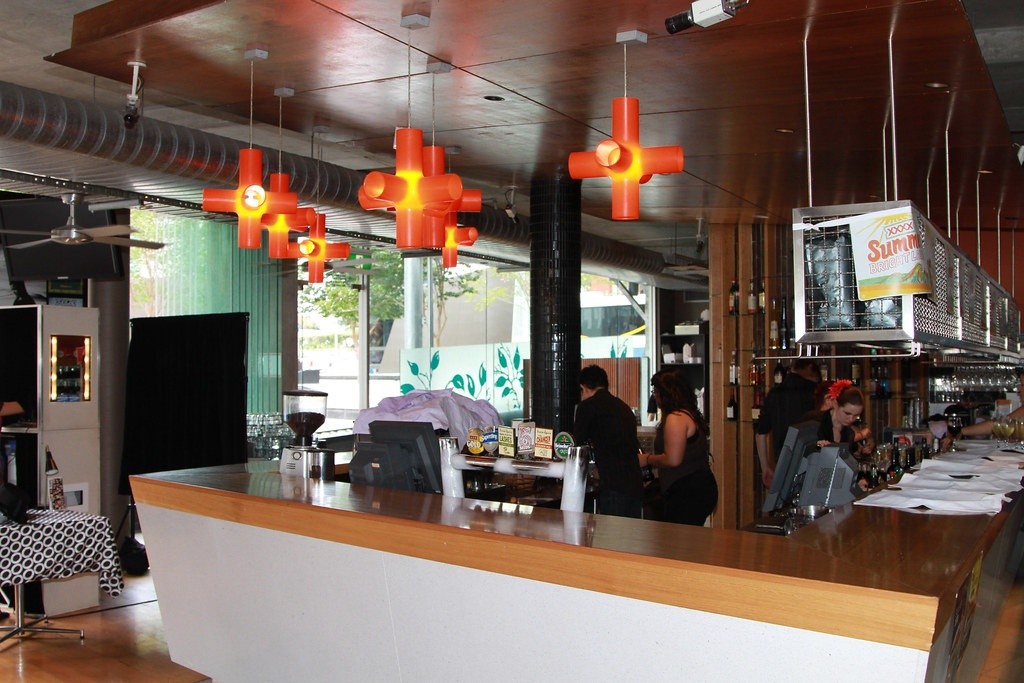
[675,320,708,336]
[661,343,682,364]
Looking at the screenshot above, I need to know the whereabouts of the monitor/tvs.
[369,421,443,495]
[762,418,820,513]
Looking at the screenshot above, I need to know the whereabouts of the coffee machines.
[280,390,334,480]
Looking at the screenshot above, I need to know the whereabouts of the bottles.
[748,286,757,313]
[726,389,737,420]
[855,464,880,488]
[820,352,828,381]
[886,449,903,481]
[774,353,784,383]
[903,447,916,468]
[851,349,860,386]
[749,354,758,385]
[757,280,765,314]
[770,297,789,350]
[728,281,739,315]
[752,388,761,419]
[870,348,891,398]
[921,437,939,458]
[246,413,292,460]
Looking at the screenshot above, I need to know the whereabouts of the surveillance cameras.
[123,107,138,125]
[665,0,735,35]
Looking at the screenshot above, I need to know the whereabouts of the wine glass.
[935,365,1018,402]
[991,413,1024,450]
[729,351,737,384]
[947,417,962,452]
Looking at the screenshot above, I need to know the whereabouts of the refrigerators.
[0,305,100,524]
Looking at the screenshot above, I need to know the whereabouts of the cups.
[872,444,921,463]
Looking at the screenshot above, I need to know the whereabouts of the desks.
[0,508,125,645]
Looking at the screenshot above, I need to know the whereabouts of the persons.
[638,367,718,526]
[570,365,645,519]
[751,360,872,493]
[939,367,1024,452]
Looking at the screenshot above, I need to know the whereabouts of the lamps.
[289,126,352,283]
[262,86,316,257]
[444,144,477,266]
[388,60,483,247]
[358,13,463,248]
[569,29,683,220]
[201,48,300,250]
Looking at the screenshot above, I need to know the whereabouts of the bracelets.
[646,454,652,464]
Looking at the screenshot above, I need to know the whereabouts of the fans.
[0,194,168,251]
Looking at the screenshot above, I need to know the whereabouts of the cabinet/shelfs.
[658,331,710,422]
